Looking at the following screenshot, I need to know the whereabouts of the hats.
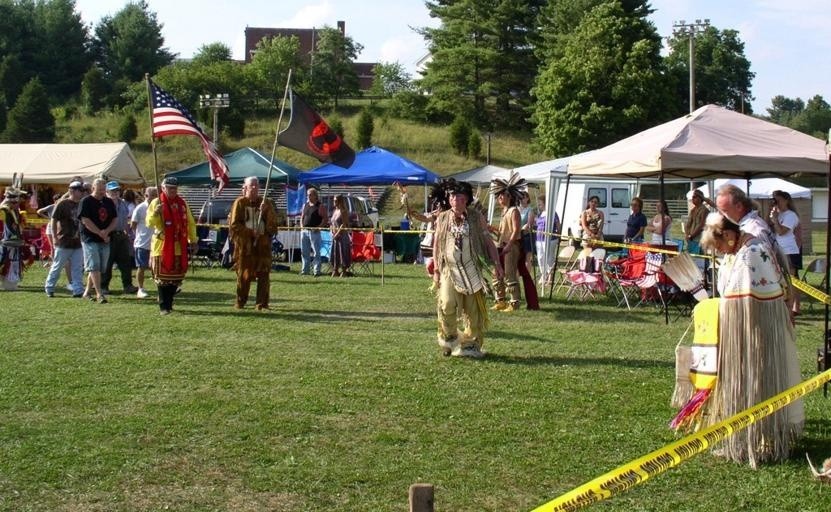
[161,177,178,187]
[105,181,121,191]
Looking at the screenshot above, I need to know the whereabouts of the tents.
[542,102,830,316]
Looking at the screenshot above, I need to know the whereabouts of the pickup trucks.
[172,192,383,269]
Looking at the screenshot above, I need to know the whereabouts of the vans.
[554,174,643,243]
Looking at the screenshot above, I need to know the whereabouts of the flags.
[149,72,228,199]
[278,88,356,171]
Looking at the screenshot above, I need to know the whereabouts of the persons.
[1,176,181,304]
[145,177,198,316]
[228,176,278,311]
[580,195,605,252]
[299,188,351,278]
[624,198,647,253]
[717,185,804,317]
[408,190,562,312]
[647,200,672,241]
[686,213,805,466]
[684,190,710,254]
[427,181,506,359]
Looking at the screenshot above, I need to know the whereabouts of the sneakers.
[46,292,53,297]
[66,283,110,304]
[137,288,149,298]
[499,302,519,312]
[489,301,506,311]
[124,285,138,294]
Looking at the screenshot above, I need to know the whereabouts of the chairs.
[544,242,828,323]
[188,228,381,278]
